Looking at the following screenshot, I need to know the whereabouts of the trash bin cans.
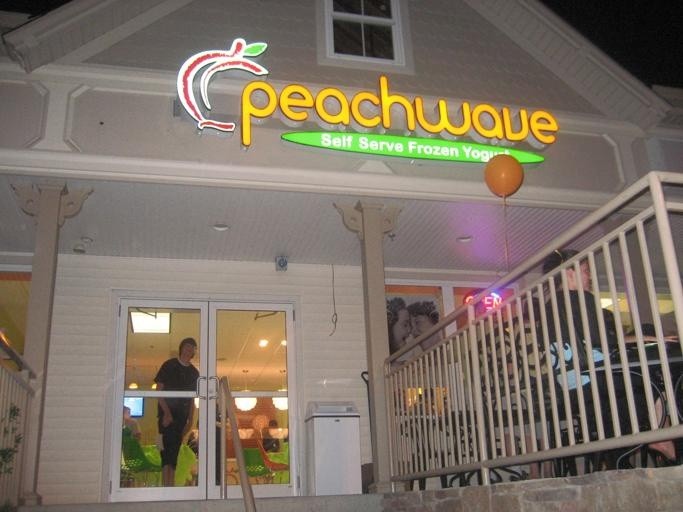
[304,402,363,496]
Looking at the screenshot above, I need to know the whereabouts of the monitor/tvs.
[124,397,144,418]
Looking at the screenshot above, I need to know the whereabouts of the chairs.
[241,447,271,484]
[255,440,290,484]
[120,424,164,488]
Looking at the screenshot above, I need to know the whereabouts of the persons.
[539,248,681,464]
[123,404,142,440]
[152,337,200,487]
[386,296,412,356]
[406,301,442,354]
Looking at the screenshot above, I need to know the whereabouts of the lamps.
[271,369,288,411]
[233,369,257,412]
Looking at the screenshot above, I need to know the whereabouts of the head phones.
[424,301,439,324]
[387,297,404,323]
